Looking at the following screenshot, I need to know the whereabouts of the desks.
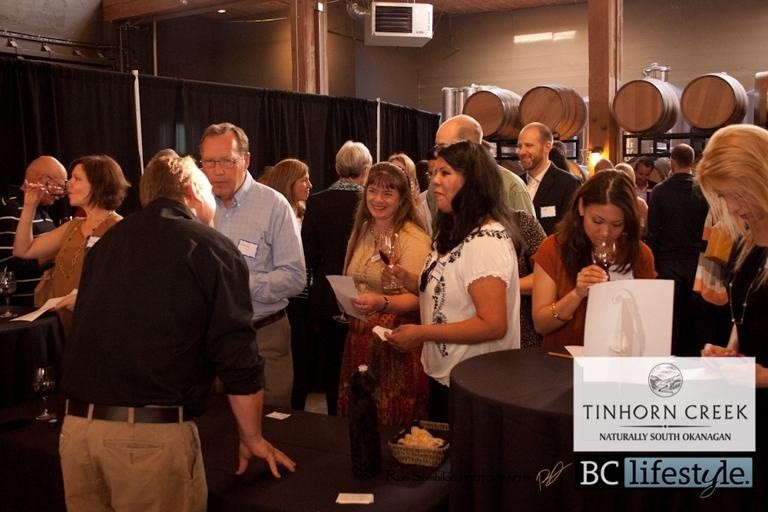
[0,305,686,512]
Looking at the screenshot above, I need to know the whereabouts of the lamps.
[588,146,608,176]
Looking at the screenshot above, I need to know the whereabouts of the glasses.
[199,155,244,172]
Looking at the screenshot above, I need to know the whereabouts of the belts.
[66,399,193,423]
[252,309,286,330]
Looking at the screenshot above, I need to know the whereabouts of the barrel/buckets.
[613,80,681,136]
[462,90,520,139]
[519,85,587,138]
[682,73,748,134]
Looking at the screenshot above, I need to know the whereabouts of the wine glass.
[0,270,20,319]
[332,309,352,324]
[29,365,58,421]
[378,230,403,291]
[58,215,73,224]
[591,237,616,281]
[23,175,72,197]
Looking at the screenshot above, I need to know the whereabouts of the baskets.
[388,418,450,468]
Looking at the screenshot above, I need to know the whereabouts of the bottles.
[347,363,383,479]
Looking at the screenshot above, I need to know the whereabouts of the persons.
[1,115,768,511]
